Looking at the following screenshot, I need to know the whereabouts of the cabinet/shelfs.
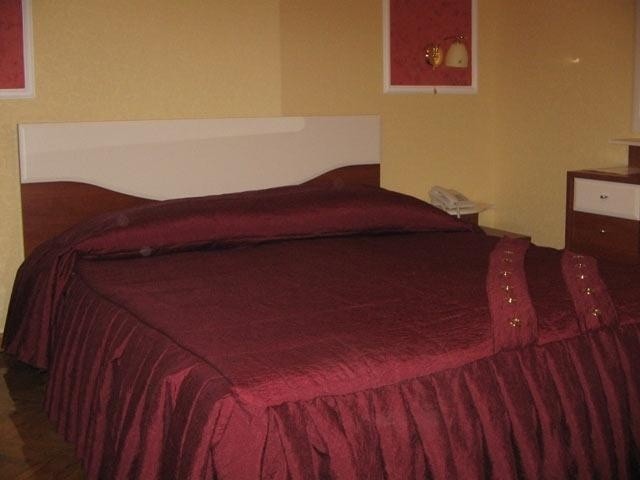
[564,137,640,276]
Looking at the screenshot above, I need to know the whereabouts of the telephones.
[428,186,474,209]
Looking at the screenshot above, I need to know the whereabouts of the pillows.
[1,185,484,370]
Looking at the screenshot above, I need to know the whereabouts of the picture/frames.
[0,0,34,99]
[382,0,478,96]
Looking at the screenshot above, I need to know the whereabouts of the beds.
[1,112,640,479]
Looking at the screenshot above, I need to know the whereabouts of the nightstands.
[448,213,532,243]
